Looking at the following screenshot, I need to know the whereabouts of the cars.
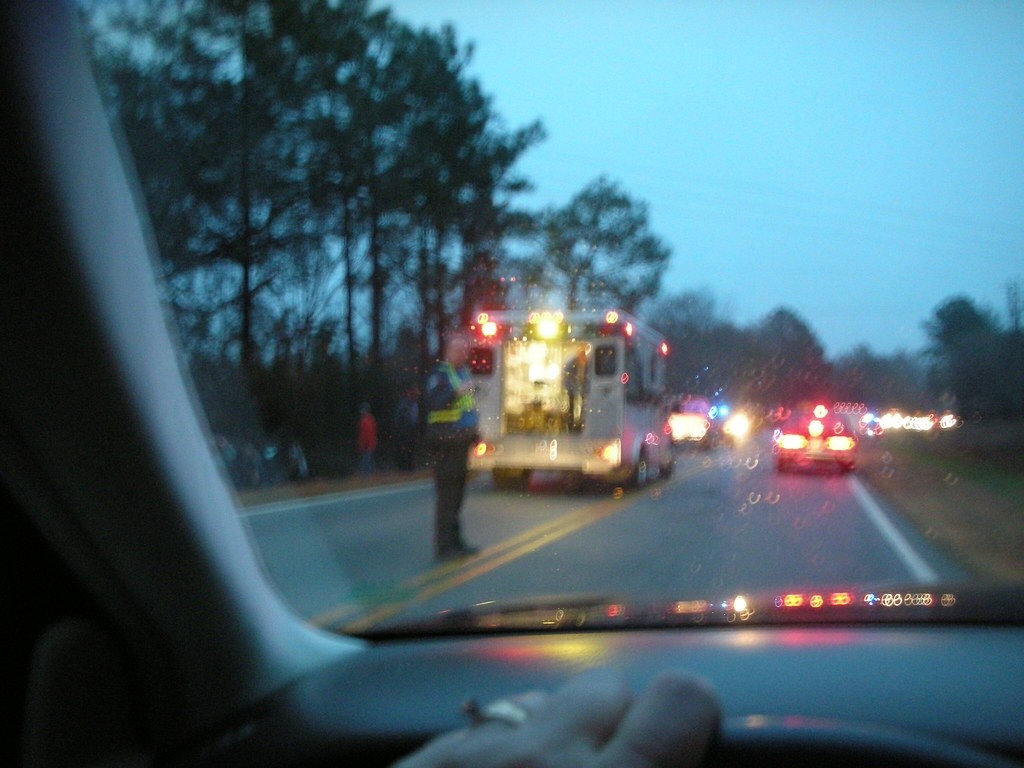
[665,391,962,477]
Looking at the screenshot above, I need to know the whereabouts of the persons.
[392,671,721,768]
[422,331,481,559]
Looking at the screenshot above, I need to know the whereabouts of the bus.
[464,306,677,489]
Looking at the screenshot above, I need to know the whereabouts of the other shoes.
[455,537,478,554]
[434,546,464,559]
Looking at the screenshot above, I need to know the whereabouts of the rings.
[461,695,530,729]
